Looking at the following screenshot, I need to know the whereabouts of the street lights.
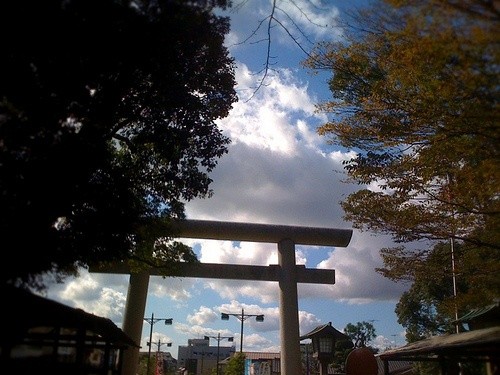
[147,339,172,352]
[193,351,212,375]
[204,333,234,375]
[300,322,351,375]
[221,308,264,352]
[143,312,174,375]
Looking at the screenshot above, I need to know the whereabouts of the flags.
[165,319,172,325]
[221,315,228,319]
[256,316,264,322]
[147,342,151,346]
[167,343,172,348]
[228,337,234,342]
[204,337,209,340]
